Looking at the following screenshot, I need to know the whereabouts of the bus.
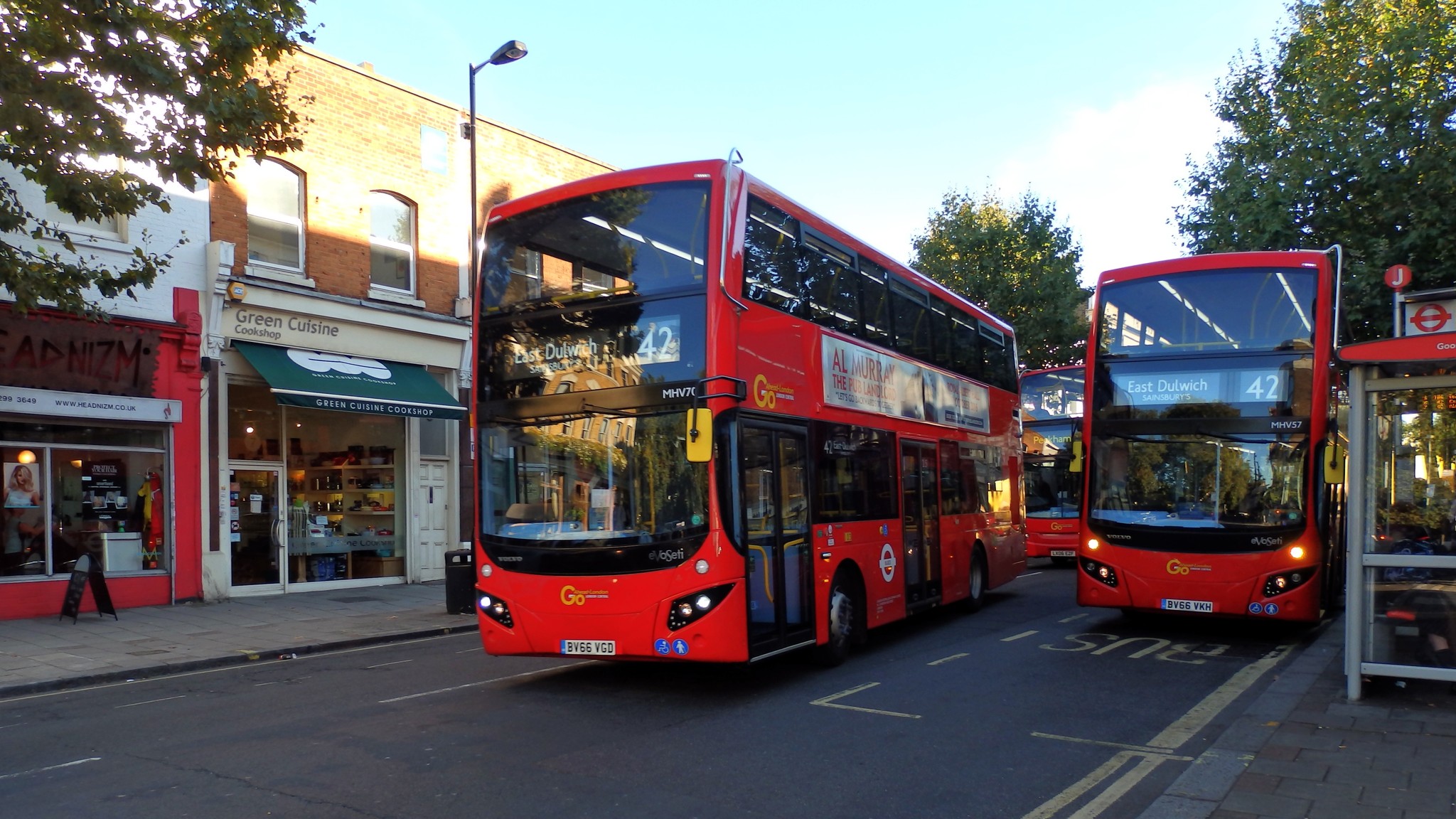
[1019,364,1086,565]
[1068,244,1348,624]
[473,147,1029,665]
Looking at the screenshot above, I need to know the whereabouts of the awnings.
[232,339,468,422]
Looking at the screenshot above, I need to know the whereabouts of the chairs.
[744,280,1014,390]
[905,496,958,527]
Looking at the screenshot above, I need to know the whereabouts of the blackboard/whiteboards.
[61,552,116,618]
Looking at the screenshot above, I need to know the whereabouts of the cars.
[1376,524,1433,555]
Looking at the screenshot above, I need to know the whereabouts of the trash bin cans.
[444,548,477,613]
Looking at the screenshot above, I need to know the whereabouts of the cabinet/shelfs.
[235,450,405,577]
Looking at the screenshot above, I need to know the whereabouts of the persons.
[3,465,40,507]
[0,509,44,575]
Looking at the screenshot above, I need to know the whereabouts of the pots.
[348,476,361,484]
[367,484,385,489]
[314,500,324,511]
[377,528,394,535]
[371,505,388,511]
[347,506,361,511]
[368,457,386,465]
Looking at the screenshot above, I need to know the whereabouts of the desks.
[82,532,143,571]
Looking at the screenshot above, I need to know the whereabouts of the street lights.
[469,40,581,612]
